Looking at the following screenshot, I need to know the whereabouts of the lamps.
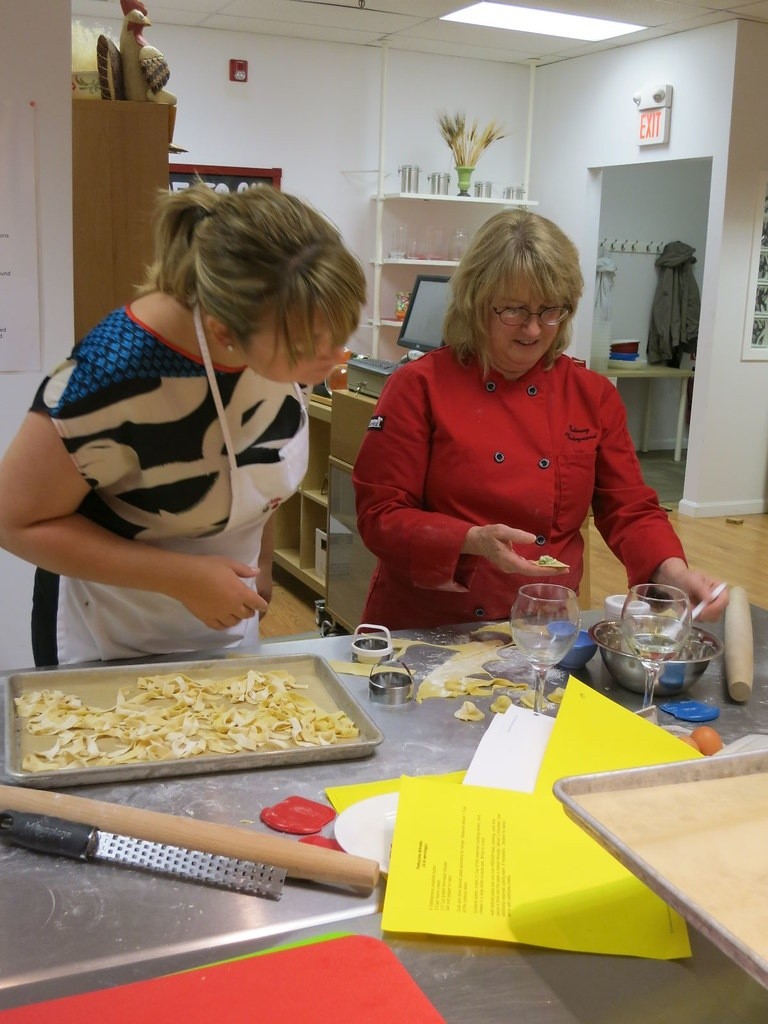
[633,84,673,146]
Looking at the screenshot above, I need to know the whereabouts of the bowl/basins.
[589,615,724,697]
[610,339,640,353]
[557,631,598,669]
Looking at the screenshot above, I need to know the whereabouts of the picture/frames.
[740,169,768,361]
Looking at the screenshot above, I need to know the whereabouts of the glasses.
[492,302,573,326]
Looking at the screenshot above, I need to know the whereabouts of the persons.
[352,207,729,635]
[1,167,368,668]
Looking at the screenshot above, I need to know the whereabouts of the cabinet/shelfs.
[73,101,174,347]
[271,382,379,636]
[368,194,540,328]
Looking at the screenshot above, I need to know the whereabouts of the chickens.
[97,0,177,106]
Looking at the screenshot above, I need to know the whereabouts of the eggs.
[676,725,724,756]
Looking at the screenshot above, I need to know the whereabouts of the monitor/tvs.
[396,274,455,351]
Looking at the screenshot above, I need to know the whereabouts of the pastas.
[13,666,360,775]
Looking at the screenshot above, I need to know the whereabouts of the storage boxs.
[591,319,648,372]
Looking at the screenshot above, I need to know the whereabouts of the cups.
[395,292,412,321]
[408,228,472,262]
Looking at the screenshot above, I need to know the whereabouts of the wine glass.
[511,584,580,715]
[621,584,692,710]
[387,223,407,260]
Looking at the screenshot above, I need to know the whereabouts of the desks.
[592,364,695,462]
[0,579,768,1024]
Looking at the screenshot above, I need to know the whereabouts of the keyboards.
[344,356,402,376]
